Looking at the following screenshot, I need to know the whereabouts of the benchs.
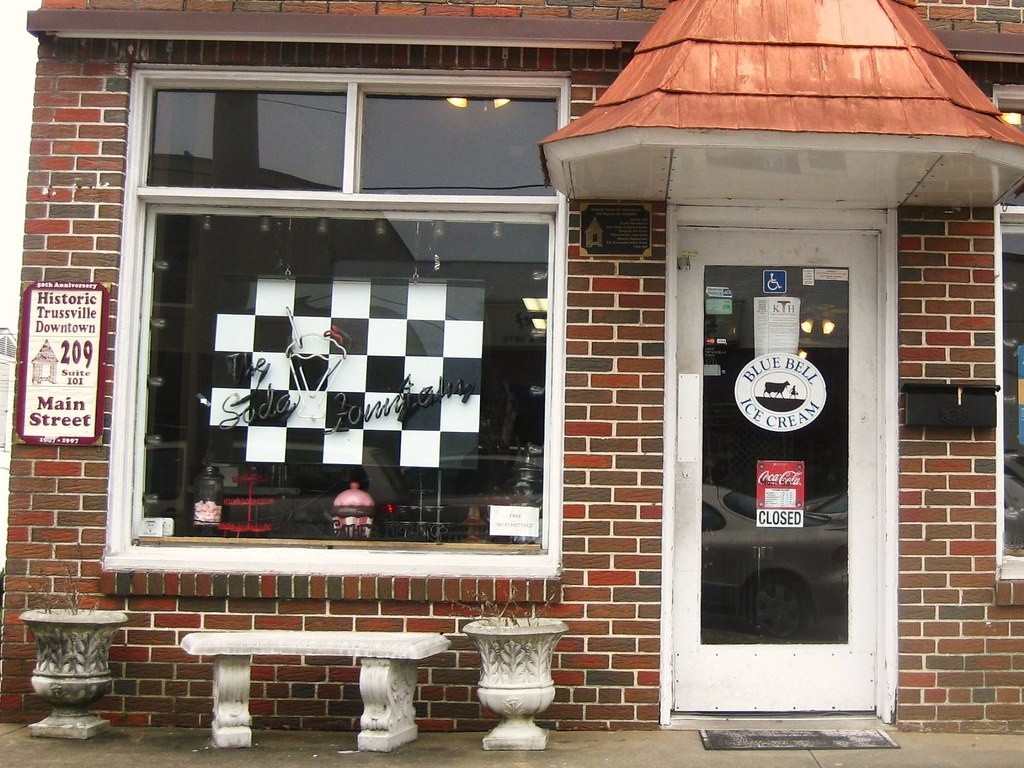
[179,633,451,753]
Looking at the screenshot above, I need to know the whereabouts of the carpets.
[696,728,901,752]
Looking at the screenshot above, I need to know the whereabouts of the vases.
[463,617,569,751]
[18,609,129,739]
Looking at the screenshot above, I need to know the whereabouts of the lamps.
[800,312,835,336]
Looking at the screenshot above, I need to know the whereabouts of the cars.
[217,441,410,538]
[395,445,543,523]
[701,468,848,639]
